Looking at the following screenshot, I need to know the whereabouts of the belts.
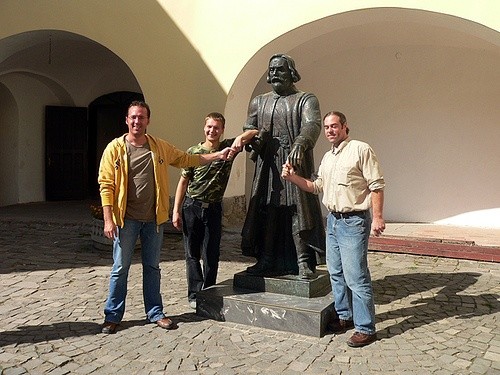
[330,211,366,219]
[184,196,221,208]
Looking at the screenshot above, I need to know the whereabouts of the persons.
[98,102,236,333]
[171,111,258,309]
[281,110,385,347]
[243,53,322,279]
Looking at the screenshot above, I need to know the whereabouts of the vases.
[90,216,142,252]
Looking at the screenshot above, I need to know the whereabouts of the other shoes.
[188,300,196,309]
[102,321,118,333]
[347,332,377,347]
[328,319,355,334]
[157,317,177,329]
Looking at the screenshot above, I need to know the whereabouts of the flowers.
[90,189,103,220]
